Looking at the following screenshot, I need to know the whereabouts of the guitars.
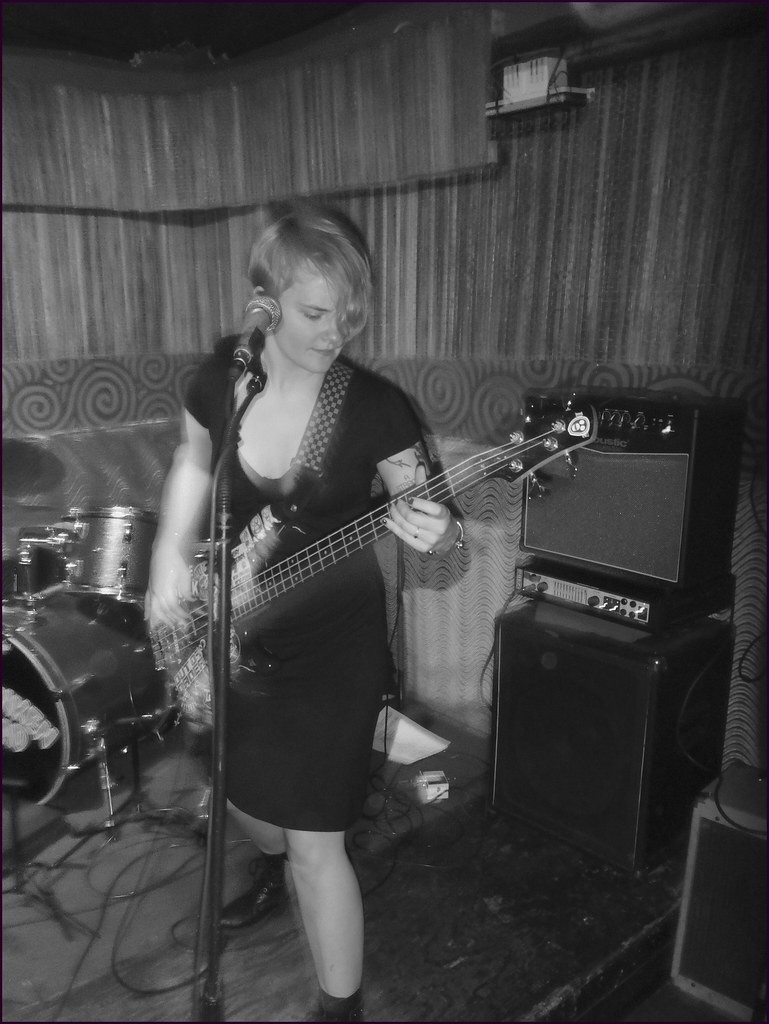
[142,386,604,735]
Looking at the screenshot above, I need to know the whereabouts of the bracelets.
[456,519,465,549]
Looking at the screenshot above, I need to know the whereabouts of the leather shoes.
[217,858,287,935]
[304,999,364,1022]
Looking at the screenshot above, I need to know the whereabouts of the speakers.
[517,384,744,593]
[670,758,769,1022]
[488,596,737,875]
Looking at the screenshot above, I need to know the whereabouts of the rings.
[414,526,419,539]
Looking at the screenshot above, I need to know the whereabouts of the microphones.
[228,293,283,384]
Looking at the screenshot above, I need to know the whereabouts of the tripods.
[1,723,207,943]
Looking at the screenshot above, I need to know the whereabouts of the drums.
[64,505,160,607]
[1,580,172,807]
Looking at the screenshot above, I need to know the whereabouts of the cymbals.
[0,505,69,530]
[0,434,67,498]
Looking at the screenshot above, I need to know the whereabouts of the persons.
[145,208,460,1024]
